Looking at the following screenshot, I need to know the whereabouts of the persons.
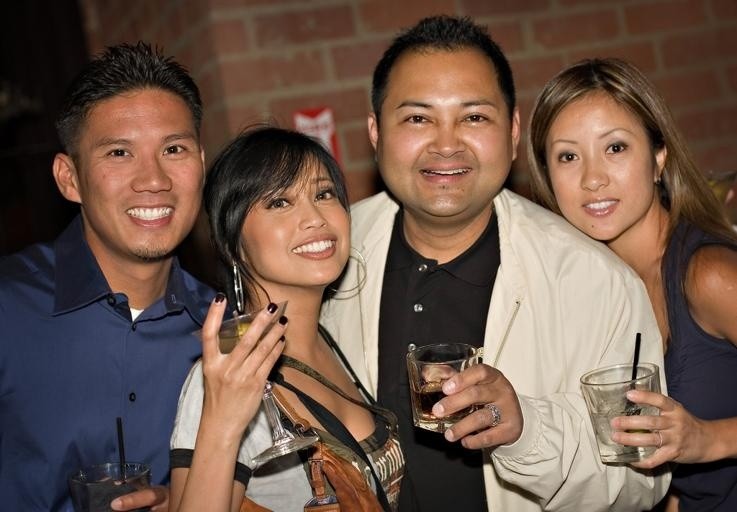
[165,117,406,512]
[524,54,737,512]
[317,11,675,511]
[0,39,234,511]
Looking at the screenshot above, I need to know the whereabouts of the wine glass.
[190,300,321,468]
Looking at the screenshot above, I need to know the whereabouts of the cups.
[68,460,155,511]
[579,360,664,464]
[405,341,479,435]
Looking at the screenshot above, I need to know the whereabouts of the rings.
[484,403,501,426]
[654,430,662,448]
